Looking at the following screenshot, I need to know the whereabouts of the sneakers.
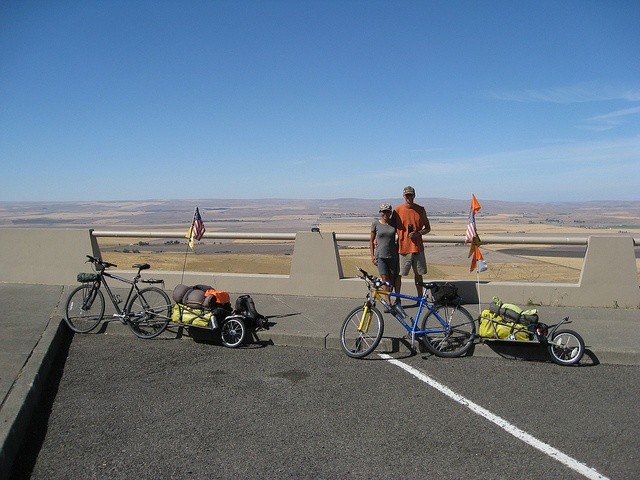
[393,299,401,308]
[383,304,392,313]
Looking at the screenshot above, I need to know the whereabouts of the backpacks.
[234,294,269,332]
[478,296,539,340]
[170,301,214,329]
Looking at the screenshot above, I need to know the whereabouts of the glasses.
[378,203,393,213]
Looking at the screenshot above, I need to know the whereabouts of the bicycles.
[64,255,175,339]
[339,267,476,358]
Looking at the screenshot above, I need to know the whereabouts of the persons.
[370,203,396,313]
[392,186,431,307]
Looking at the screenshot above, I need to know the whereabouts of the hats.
[402,185,415,197]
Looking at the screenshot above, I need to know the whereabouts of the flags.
[464,210,477,245]
[191,207,206,242]
